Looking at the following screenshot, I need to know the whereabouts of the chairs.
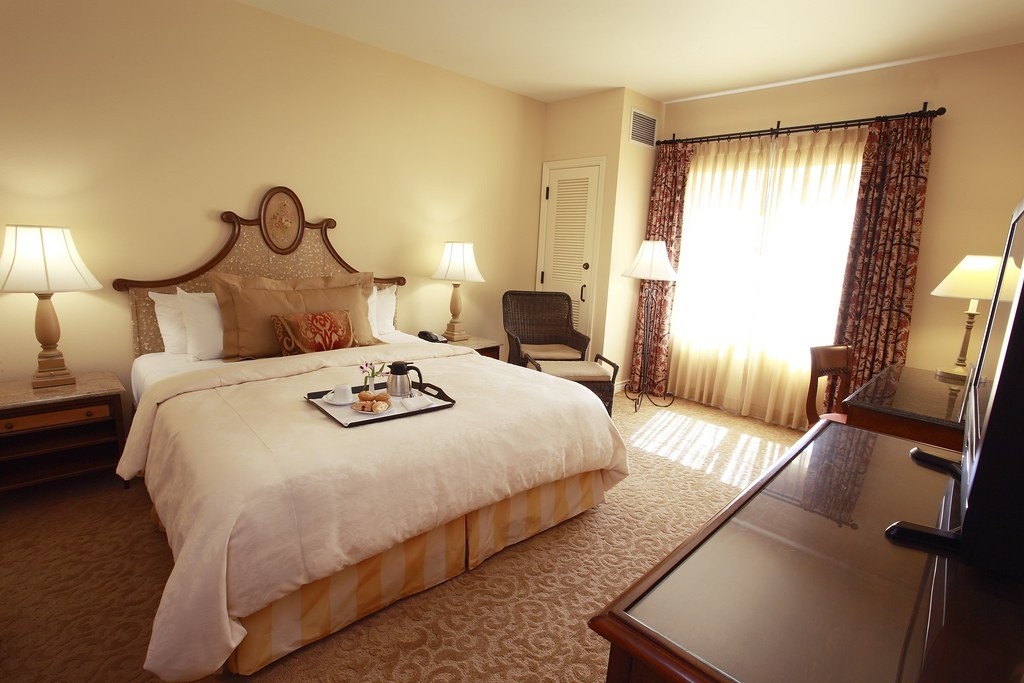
[502,289,591,367]
[806,346,853,427]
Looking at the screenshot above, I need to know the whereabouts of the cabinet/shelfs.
[585,198,1024,683]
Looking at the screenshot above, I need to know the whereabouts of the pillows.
[148,271,398,363]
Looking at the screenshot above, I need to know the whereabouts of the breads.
[352,390,392,413]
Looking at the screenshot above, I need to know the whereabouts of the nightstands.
[0,370,128,504]
[447,337,504,363]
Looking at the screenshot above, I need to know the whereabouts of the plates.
[350,404,394,414]
[322,393,359,405]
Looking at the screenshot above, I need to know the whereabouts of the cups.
[332,384,352,402]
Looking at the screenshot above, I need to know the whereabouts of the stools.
[524,352,618,421]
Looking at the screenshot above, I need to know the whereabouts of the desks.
[842,363,962,450]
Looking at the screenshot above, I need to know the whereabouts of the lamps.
[929,254,1001,379]
[620,240,683,413]
[0,223,103,387]
[430,241,486,342]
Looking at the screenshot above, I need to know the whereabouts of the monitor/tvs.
[958,198,1024,543]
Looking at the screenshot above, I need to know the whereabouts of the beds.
[113,186,629,679]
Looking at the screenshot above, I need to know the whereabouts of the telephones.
[418,330,448,343]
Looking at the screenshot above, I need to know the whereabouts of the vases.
[369,378,375,393]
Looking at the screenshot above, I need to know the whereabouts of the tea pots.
[386,361,422,396]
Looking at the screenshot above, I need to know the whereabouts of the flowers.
[360,361,386,388]
